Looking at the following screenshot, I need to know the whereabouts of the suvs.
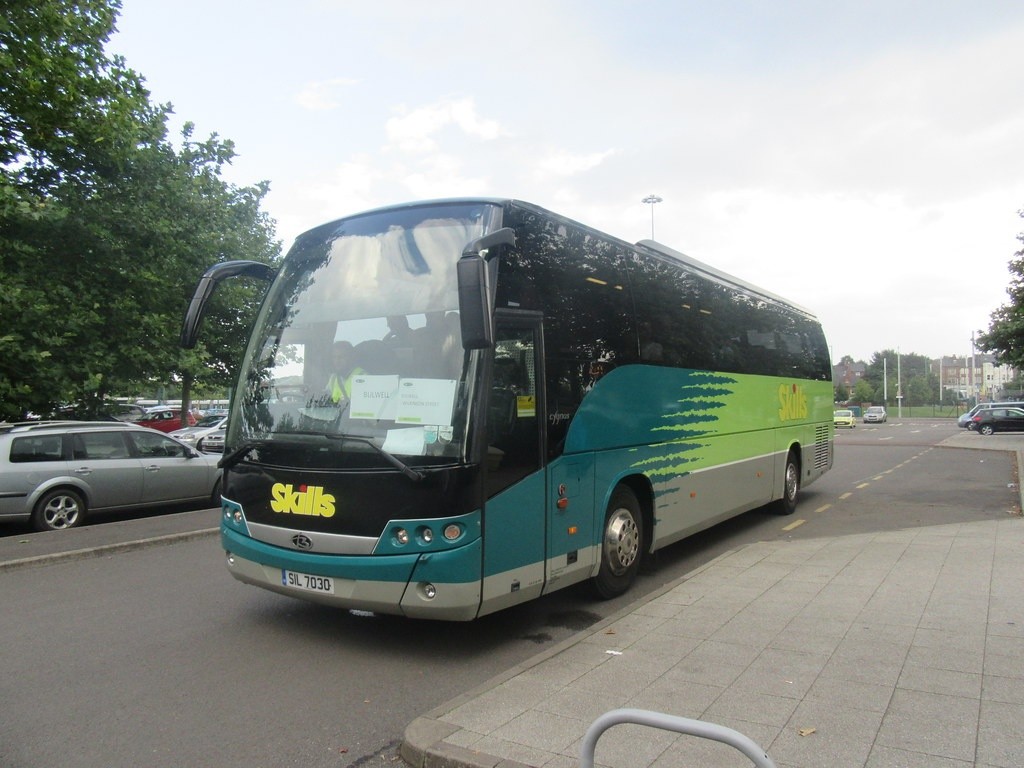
[0,421,223,533]
[969,407,1024,436]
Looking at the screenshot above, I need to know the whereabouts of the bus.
[176,196,835,624]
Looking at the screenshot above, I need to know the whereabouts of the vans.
[957,402,1024,432]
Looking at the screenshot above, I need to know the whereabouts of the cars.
[863,406,887,424]
[834,410,856,429]
[26,403,229,455]
